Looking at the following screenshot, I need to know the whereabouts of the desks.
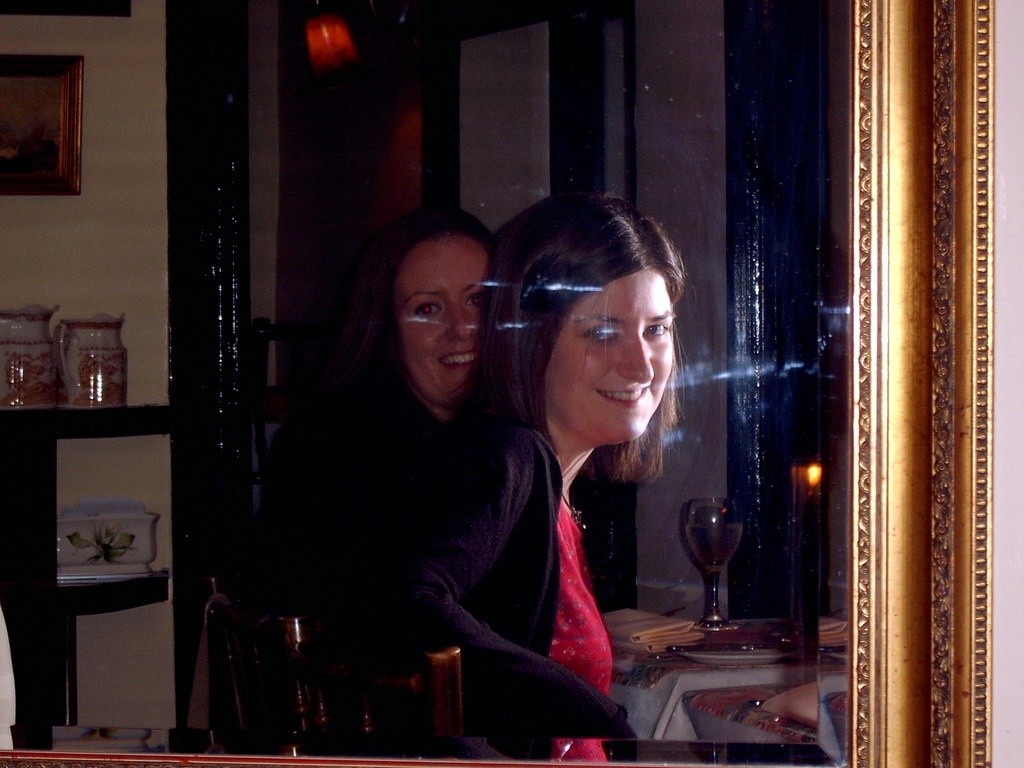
[584,617,847,765]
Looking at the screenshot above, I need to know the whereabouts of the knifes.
[665,644,775,650]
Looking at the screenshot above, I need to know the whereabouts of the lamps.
[304,2,359,70]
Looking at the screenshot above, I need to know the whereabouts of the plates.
[676,649,784,665]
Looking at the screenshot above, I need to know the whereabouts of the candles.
[798,463,830,662]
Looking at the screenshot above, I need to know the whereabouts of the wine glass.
[679,497,742,632]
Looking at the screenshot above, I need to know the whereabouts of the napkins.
[595,609,847,728]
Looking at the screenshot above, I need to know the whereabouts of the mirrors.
[0,1,995,768]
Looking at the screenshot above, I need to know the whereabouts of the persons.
[258,190,685,741]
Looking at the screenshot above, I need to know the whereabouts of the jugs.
[51,312,128,404]
[0,304,58,410]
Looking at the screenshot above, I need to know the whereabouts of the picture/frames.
[0,54,84,197]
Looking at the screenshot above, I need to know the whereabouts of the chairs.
[188,577,464,757]
